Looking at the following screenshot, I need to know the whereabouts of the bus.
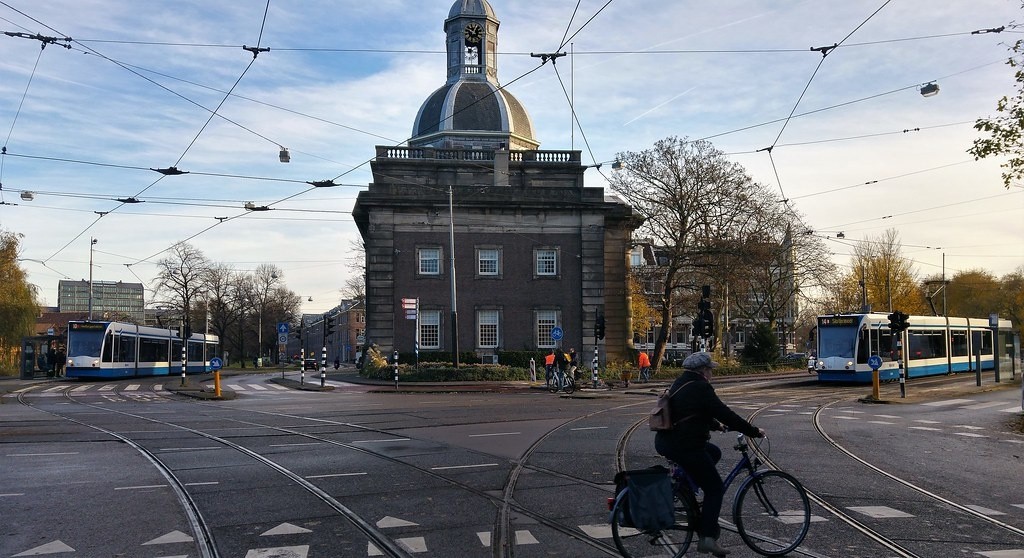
[65,314,217,378]
[808,280,1013,384]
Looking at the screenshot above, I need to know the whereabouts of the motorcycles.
[334,362,340,370]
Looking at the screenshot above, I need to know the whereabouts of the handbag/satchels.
[614,465,675,533]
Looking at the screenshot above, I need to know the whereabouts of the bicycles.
[545,366,576,394]
[605,426,812,558]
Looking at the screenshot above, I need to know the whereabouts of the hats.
[683,352,718,369]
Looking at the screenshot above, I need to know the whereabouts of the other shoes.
[697,537,731,555]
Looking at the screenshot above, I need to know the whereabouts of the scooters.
[806,352,818,374]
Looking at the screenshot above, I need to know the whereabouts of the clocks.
[463,21,484,43]
[464,45,478,60]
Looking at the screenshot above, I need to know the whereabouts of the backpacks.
[649,379,701,431]
[559,352,572,363]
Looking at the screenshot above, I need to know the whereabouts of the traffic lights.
[888,310,899,329]
[702,310,712,336]
[900,311,910,330]
[176,325,184,339]
[296,328,302,340]
[325,316,336,338]
[597,315,608,340]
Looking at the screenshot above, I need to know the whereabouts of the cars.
[785,353,805,361]
[304,359,319,372]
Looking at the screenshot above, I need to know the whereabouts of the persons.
[545,347,579,392]
[37,347,66,378]
[253,356,258,370]
[637,352,651,383]
[654,352,765,555]
[334,357,339,368]
